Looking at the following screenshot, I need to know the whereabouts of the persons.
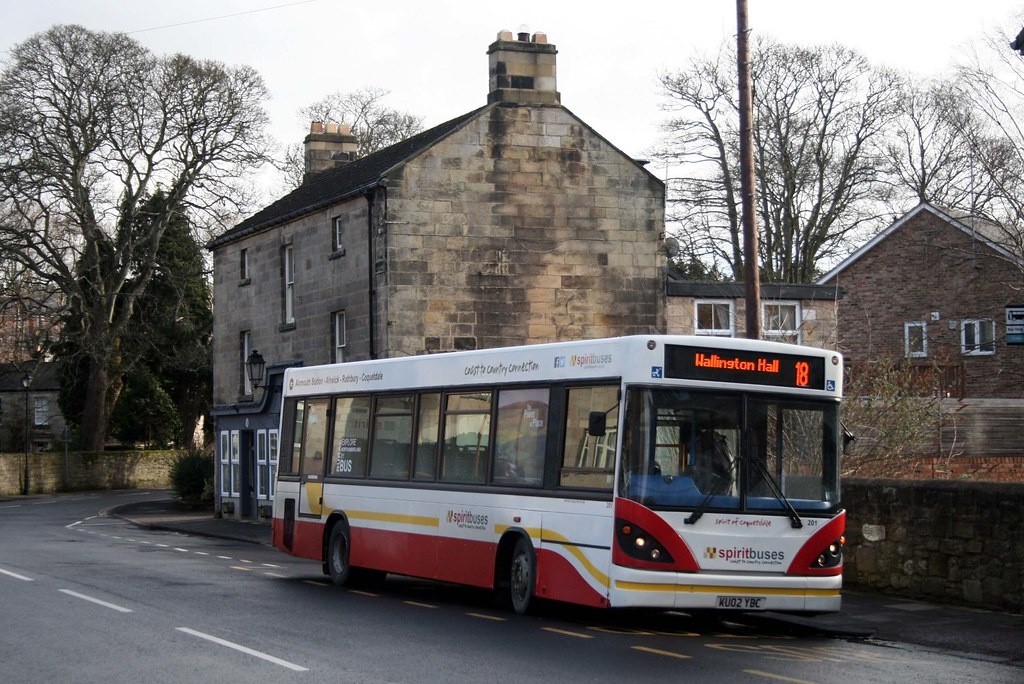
[622,420,634,472]
[679,425,734,495]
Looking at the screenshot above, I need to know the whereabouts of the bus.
[270,336,859,625]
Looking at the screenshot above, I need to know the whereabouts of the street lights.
[21,373,35,495]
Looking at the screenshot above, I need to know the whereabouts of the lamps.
[243,350,283,394]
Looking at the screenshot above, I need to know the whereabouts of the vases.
[221,501,234,514]
[259,504,272,519]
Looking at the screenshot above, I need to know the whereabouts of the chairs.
[342,436,513,483]
[632,461,662,479]
